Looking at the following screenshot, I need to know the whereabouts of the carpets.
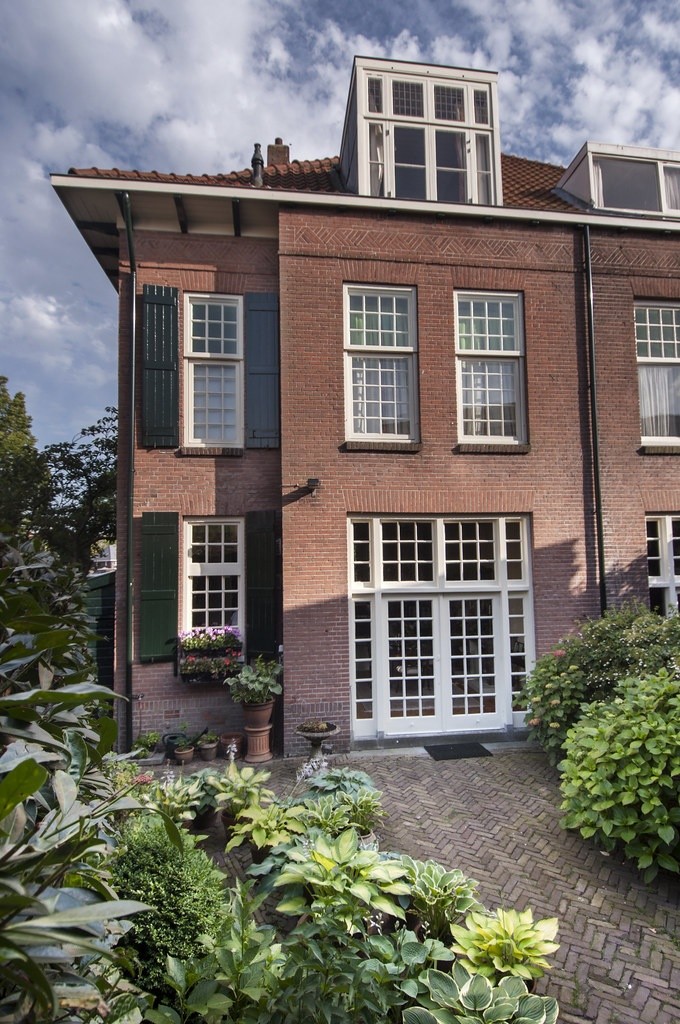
[424,742,493,761]
[357,681,480,712]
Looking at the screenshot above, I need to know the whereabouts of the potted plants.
[185,768,225,829]
[175,722,194,765]
[197,729,220,761]
[335,786,389,852]
[223,654,284,728]
[295,722,340,762]
[354,855,561,1024]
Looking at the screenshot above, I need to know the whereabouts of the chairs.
[395,644,430,696]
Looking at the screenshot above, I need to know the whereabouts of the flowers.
[209,738,331,855]
[177,625,243,678]
[273,829,412,945]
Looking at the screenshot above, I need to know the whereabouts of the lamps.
[307,477,321,494]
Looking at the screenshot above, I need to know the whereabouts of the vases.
[221,804,252,846]
[221,732,245,760]
[249,837,274,880]
[297,913,371,956]
